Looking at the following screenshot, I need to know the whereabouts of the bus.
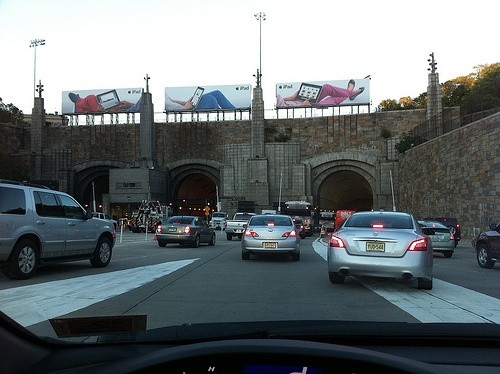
[282,201,315,237]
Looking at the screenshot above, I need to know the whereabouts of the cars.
[327,211,433,289]
[156,215,216,248]
[242,215,300,261]
[418,220,455,257]
[471,222,500,269]
[292,218,305,239]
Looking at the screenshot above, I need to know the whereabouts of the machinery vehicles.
[131,199,173,233]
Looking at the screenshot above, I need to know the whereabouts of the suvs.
[0,180,116,280]
[420,218,461,248]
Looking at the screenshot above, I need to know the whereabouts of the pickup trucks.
[93,213,117,232]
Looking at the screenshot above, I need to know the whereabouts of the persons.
[165,90,237,110]
[68,93,143,113]
[277,79,364,106]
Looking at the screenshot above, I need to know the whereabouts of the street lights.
[255,12,266,88]
[29,38,46,98]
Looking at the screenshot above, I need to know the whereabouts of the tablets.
[96,89,120,110]
[190,87,205,110]
[298,83,323,104]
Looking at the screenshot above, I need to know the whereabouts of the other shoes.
[348,79,355,91]
[350,86,365,100]
[69,93,80,103]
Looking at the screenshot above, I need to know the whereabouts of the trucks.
[225,213,256,240]
[209,212,227,229]
